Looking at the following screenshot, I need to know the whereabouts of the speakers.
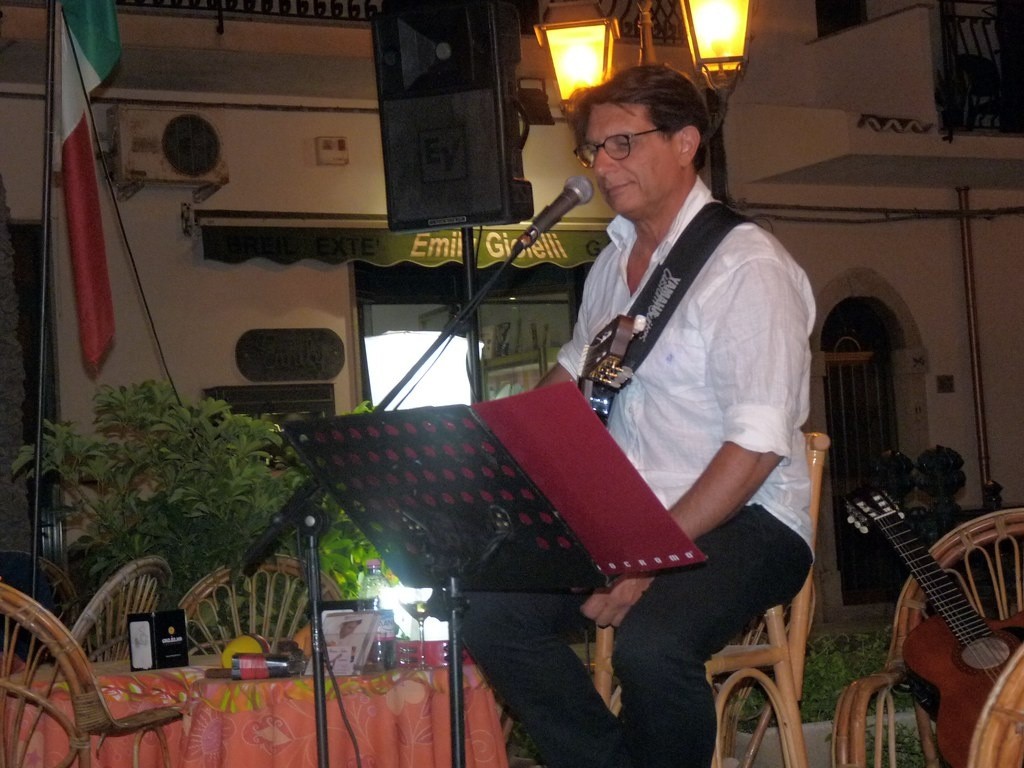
[371,0,535,235]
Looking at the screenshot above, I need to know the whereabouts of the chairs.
[591,432,831,767]
[832,508,1023,768]
[0,548,347,767]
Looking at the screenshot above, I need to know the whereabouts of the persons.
[451,64,816,768]
[323,613,362,647]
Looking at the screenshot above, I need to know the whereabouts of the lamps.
[516,77,555,126]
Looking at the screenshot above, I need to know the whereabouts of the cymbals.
[391,639,478,669]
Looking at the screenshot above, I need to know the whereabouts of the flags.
[61,0,121,363]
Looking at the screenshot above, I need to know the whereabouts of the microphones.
[519,177,595,249]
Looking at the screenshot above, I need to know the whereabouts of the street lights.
[524,0,748,192]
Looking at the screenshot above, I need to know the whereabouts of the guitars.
[574,314,649,425]
[842,487,1024,768]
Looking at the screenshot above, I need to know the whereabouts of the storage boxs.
[127,609,189,672]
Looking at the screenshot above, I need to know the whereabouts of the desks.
[0,653,509,767]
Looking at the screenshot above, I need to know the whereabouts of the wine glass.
[398,584,439,671]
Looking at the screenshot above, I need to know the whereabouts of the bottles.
[358,560,397,671]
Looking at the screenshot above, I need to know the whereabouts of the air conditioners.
[106,105,229,189]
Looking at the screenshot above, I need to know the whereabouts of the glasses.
[572,127,675,171]
